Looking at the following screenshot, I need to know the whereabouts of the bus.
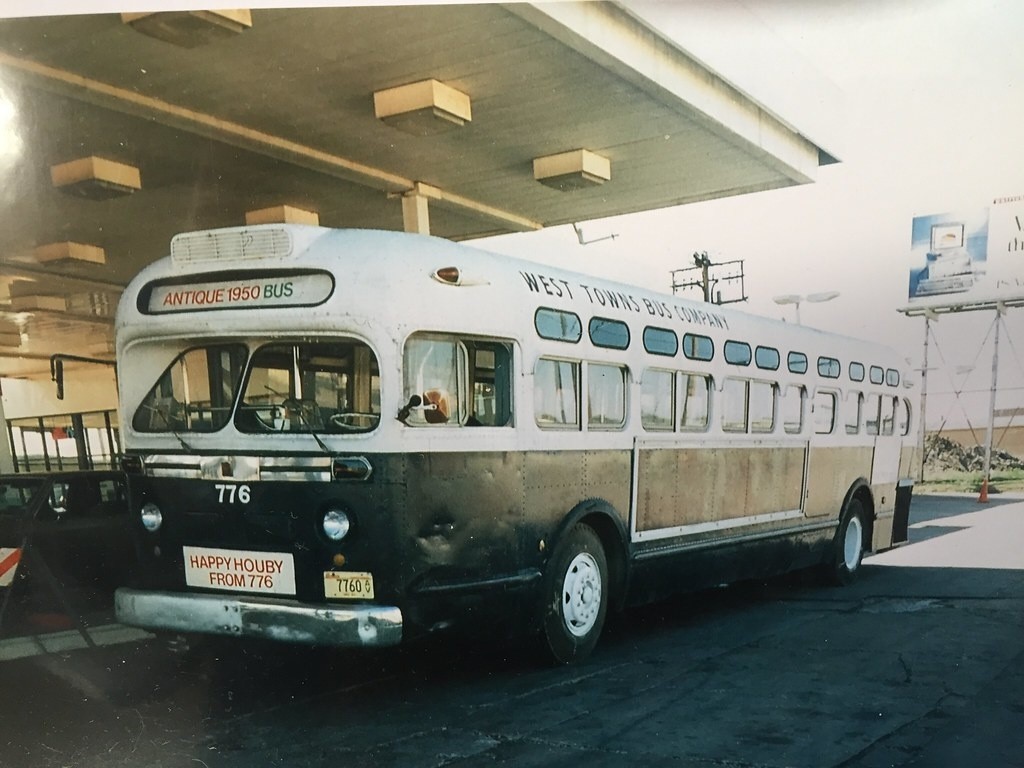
[49,221,923,666]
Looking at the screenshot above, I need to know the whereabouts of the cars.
[0,469,128,623]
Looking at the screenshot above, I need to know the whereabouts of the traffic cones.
[974,480,991,504]
